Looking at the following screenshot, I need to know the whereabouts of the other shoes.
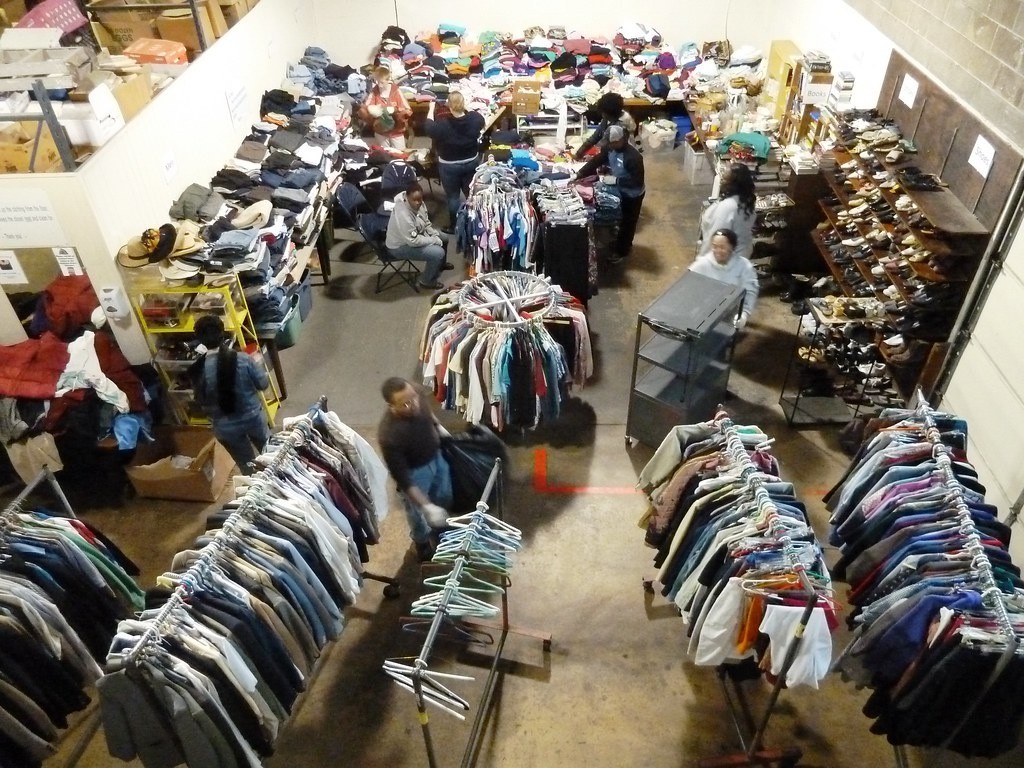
[441,262,454,270]
[792,107,962,408]
[419,282,444,289]
[441,225,454,234]
[416,542,434,562]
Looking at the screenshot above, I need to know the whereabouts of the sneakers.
[608,253,626,264]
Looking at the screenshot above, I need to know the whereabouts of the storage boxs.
[585,112,715,187]
[512,81,542,115]
[126,426,235,502]
[274,267,312,349]
[0,0,261,172]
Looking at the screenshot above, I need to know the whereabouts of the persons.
[688,228,759,329]
[366,68,412,152]
[385,182,454,290]
[566,91,646,265]
[378,377,456,560]
[91,305,148,414]
[427,91,485,236]
[697,163,757,260]
[188,315,272,476]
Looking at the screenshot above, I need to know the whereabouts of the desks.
[625,97,717,174]
[292,177,344,284]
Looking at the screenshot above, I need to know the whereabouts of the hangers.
[880,399,1024,655]
[710,411,847,611]
[0,497,34,550]
[383,646,475,721]
[126,408,323,662]
[538,183,589,228]
[456,269,568,339]
[474,155,524,205]
[402,501,522,646]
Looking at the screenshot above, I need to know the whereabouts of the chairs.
[381,162,415,201]
[336,182,390,264]
[357,213,422,294]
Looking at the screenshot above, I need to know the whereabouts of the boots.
[778,274,810,303]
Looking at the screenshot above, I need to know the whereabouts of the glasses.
[394,395,420,408]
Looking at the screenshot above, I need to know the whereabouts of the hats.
[117,221,205,268]
[597,125,626,146]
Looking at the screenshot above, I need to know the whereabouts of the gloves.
[437,424,451,436]
[423,503,449,527]
[603,175,616,185]
[734,312,749,328]
[567,174,577,185]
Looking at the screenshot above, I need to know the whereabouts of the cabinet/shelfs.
[516,114,583,139]
[761,40,836,152]
[780,112,993,430]
[130,275,281,428]
[626,268,746,452]
